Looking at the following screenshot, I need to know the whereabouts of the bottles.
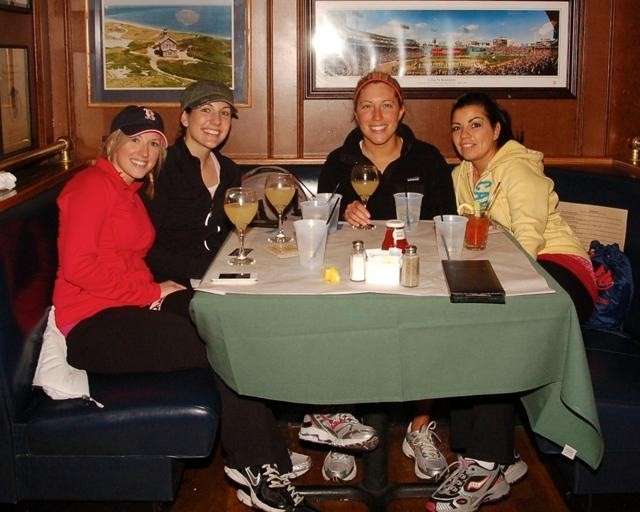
[381,221,409,251]
[348,238,367,282]
[402,244,420,288]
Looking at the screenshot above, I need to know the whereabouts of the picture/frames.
[0,44,35,160]
[0,1,33,15]
[301,1,580,100]
[83,0,253,112]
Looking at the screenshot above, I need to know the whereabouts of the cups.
[301,200,332,220]
[292,218,330,270]
[463,212,494,249]
[432,214,468,260]
[393,191,425,231]
[314,189,344,234]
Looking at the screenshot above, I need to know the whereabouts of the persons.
[316,70,460,485]
[151,78,383,483]
[422,92,596,512]
[52,105,323,511]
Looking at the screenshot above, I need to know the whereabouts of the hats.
[181,80,238,113]
[111,106,168,148]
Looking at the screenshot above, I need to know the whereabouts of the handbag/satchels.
[585,241,633,329]
[241,166,308,226]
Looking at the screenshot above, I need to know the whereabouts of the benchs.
[1,136,639,512]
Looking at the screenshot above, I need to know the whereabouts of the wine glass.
[222,185,260,268]
[347,162,381,230]
[262,172,297,244]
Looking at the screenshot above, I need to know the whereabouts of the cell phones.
[227,246,253,260]
[210,272,259,285]
